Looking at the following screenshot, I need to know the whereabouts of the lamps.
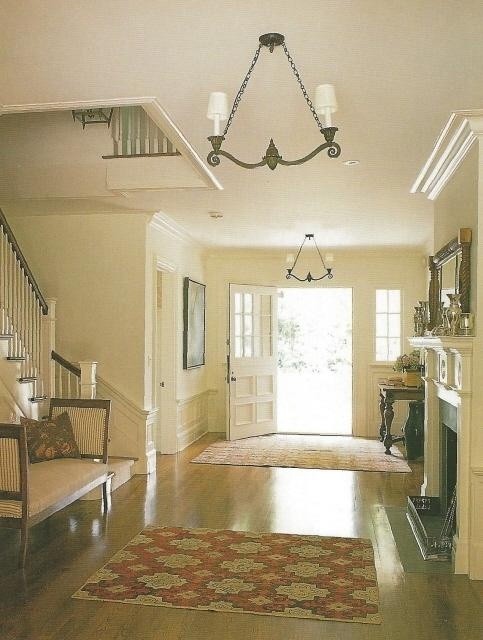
[207,32,342,171]
[71,110,113,128]
[285,232,336,284]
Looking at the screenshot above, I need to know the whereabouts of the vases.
[404,372,421,387]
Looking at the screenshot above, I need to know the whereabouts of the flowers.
[394,350,420,370]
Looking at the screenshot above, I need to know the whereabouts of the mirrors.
[430,228,472,334]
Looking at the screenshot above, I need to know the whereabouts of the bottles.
[414,294,462,335]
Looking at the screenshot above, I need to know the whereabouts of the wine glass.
[459,312,475,337]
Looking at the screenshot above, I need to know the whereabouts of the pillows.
[19,411,82,465]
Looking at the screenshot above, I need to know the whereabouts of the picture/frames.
[182,276,207,370]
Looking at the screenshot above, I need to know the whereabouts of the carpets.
[71,523,384,627]
[189,434,414,473]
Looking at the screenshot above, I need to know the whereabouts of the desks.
[377,384,424,455]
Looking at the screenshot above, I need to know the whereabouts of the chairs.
[0,397,114,569]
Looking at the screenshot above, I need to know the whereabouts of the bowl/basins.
[374,376,402,386]
